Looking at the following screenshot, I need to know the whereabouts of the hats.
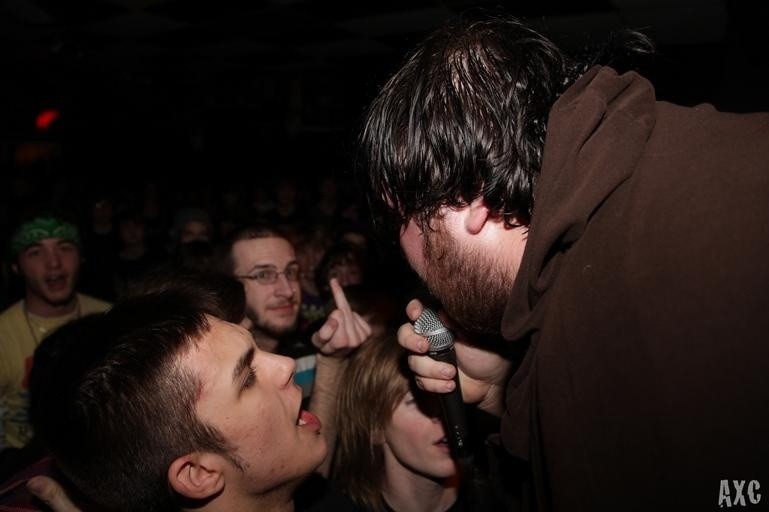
[5,206,83,255]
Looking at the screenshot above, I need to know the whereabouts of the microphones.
[411,309,477,471]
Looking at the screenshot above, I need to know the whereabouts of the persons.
[352,15,768,512]
[0,168,493,512]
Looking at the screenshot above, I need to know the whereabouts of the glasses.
[238,259,302,286]
